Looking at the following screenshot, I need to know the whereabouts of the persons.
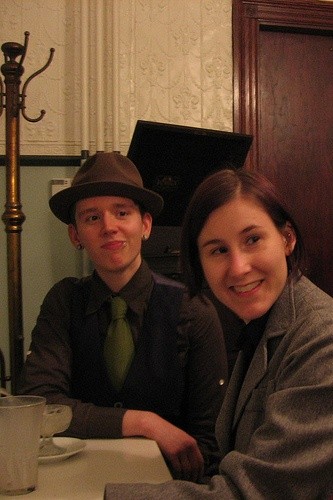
[103,168,331,500]
[20,153,230,484]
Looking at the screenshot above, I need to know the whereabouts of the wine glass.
[37,405,73,457]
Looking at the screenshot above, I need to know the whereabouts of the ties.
[103,295,136,398]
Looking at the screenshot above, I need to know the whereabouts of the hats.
[48,152,164,225]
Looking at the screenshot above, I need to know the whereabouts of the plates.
[38,437,87,465]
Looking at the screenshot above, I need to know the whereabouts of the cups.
[0,395,46,497]
